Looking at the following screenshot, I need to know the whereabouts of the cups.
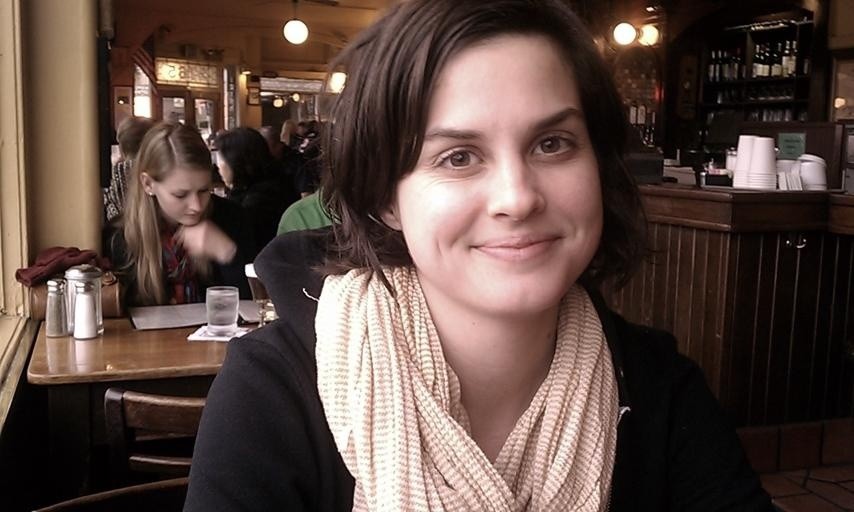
[734,133,778,191]
[204,285,241,338]
[800,161,829,191]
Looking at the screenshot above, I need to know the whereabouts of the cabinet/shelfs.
[661,9,833,127]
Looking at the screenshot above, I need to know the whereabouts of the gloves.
[16,246,97,287]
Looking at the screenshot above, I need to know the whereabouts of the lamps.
[282,0,309,45]
[638,23,659,47]
[613,22,637,46]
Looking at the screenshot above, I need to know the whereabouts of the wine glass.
[245,264,270,328]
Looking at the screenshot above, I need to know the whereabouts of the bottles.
[43,277,69,338]
[747,106,793,123]
[708,39,798,79]
[72,282,99,341]
[64,262,106,335]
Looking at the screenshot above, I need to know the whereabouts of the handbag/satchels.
[28,272,124,321]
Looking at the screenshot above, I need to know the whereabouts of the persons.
[102,116,335,244]
[277,187,343,234]
[102,121,273,307]
[181,1,788,512]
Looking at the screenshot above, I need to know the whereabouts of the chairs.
[102,386,206,488]
[28,477,192,512]
